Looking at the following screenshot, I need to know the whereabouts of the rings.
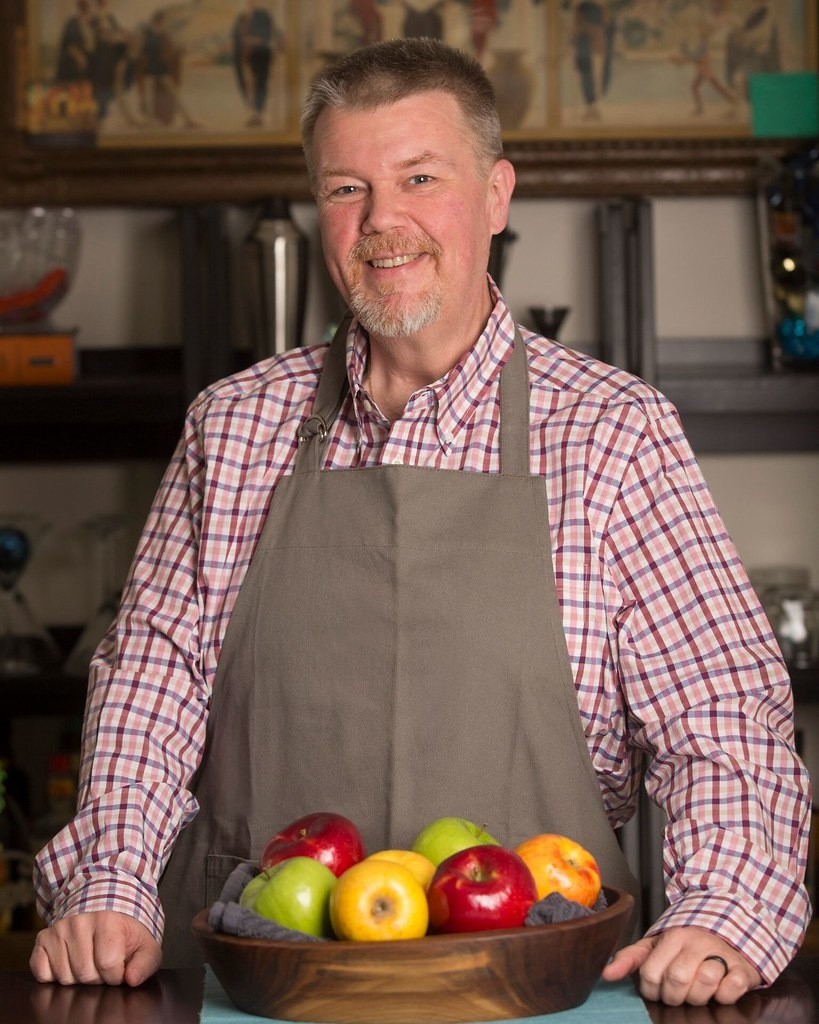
[704,955,728,976]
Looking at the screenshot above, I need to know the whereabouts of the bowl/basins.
[188,882,637,1024]
[2,209,82,336]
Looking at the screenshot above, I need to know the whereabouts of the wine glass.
[4,716,82,961]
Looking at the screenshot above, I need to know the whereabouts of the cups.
[592,192,659,390]
[749,565,819,671]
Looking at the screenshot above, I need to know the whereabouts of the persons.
[27,40,813,1007]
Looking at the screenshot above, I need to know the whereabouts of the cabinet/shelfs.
[0,170,819,884]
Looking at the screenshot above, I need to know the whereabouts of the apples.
[239,812,601,943]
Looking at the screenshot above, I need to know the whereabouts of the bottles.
[237,194,311,367]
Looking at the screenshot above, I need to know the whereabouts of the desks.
[1,925,819,1024]
